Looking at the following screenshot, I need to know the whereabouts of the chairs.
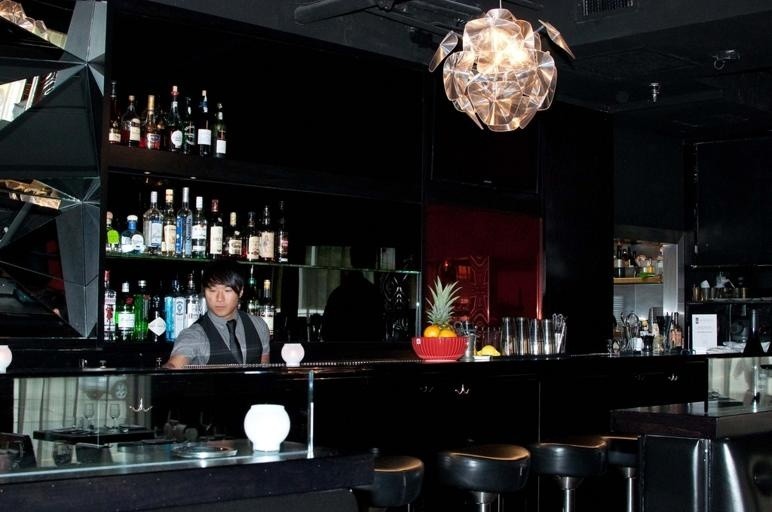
[0,432,34,450]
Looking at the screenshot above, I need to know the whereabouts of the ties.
[226,318,242,364]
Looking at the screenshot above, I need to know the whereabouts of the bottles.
[135,280,150,342]
[208,200,224,259]
[197,90,211,162]
[185,273,199,329]
[614,244,625,278]
[106,212,120,254]
[164,92,185,153]
[275,201,289,263]
[671,312,683,350]
[143,191,164,254]
[199,270,210,318]
[242,212,260,260]
[141,95,161,150]
[554,318,567,359]
[117,281,134,341]
[163,189,177,258]
[176,188,193,258]
[527,318,540,360]
[261,279,274,341]
[742,309,766,356]
[109,81,123,146]
[499,316,512,357]
[164,270,185,343]
[513,317,526,359]
[246,264,260,316]
[150,295,166,342]
[540,318,553,360]
[212,104,227,160]
[260,205,275,263]
[224,212,242,258]
[121,215,145,254]
[104,269,117,341]
[192,196,208,259]
[183,98,196,155]
[123,96,140,147]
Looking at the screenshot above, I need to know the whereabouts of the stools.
[602,435,642,512]
[438,444,531,512]
[349,454,424,512]
[533,437,608,512]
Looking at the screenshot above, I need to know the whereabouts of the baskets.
[412,336,469,360]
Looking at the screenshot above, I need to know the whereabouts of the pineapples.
[426,276,461,329]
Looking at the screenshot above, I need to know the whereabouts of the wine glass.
[109,403,121,429]
[84,402,95,430]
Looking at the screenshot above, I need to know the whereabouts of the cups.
[655,315,671,352]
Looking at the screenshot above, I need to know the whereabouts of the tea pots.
[454,320,478,361]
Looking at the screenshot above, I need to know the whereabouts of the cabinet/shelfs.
[686,295,772,358]
[614,275,664,340]
[544,100,613,352]
[428,72,545,205]
[103,0,425,347]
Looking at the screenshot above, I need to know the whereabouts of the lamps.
[429,0,578,133]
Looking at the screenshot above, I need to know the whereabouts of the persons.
[161,258,272,368]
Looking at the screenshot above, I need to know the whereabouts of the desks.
[0,436,375,512]
[611,396,772,511]
[33,426,155,445]
